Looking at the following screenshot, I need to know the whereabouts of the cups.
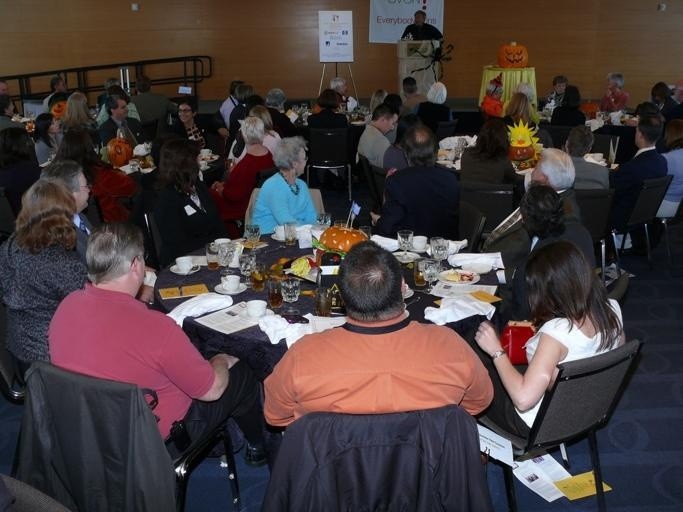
[311,287,332,317]
[197,147,219,170]
[273,222,296,245]
[175,256,197,272]
[13,108,35,131]
[595,112,605,123]
[316,212,372,238]
[455,136,467,159]
[413,234,449,297]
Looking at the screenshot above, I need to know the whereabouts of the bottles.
[95,103,100,115]
[402,32,413,41]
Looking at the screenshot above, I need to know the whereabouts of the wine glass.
[207,225,301,316]
[290,100,368,124]
[396,231,414,260]
[126,137,154,173]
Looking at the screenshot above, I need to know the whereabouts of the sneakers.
[245,443,269,468]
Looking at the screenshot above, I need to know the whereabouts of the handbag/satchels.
[502,320,536,365]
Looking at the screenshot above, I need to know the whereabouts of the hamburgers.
[316,224,369,266]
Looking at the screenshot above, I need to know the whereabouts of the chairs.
[304,125,354,195]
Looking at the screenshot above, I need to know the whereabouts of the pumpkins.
[579,99,600,119]
[51,101,67,120]
[106,139,132,166]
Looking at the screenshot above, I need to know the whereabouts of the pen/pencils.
[178,285,182,296]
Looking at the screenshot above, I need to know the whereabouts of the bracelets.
[490,351,508,360]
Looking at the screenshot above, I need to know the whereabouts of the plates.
[237,310,274,321]
[435,149,461,168]
[170,264,202,275]
[388,252,420,264]
[270,232,285,242]
[438,268,482,286]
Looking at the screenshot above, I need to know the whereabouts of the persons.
[48,220,272,468]
[262,240,494,428]
[473,241,627,439]
[2,77,359,331]
[1,178,167,388]
[400,10,443,40]
[358,74,681,332]
